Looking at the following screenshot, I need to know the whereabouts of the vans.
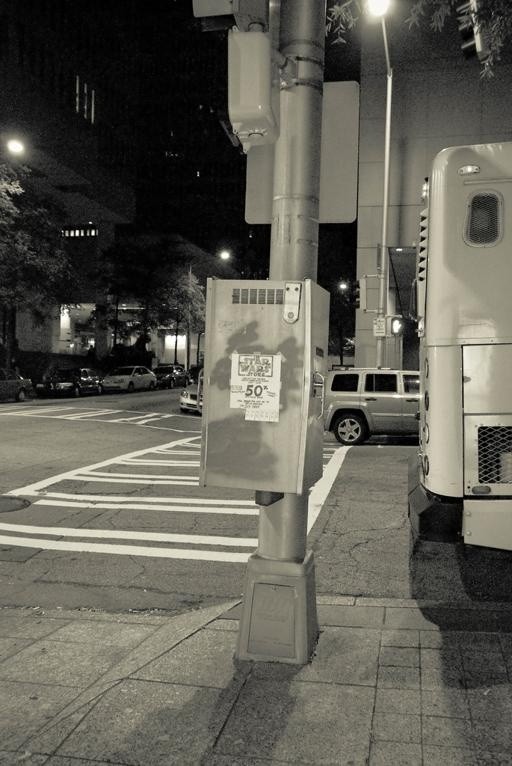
[324,368,420,445]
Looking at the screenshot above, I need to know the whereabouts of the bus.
[407,141,511,604]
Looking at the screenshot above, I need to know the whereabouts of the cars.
[100,365,204,394]
[36,366,102,396]
[0,369,32,403]
[179,379,203,413]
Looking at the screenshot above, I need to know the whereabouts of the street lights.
[339,282,347,366]
[366,0,394,365]
